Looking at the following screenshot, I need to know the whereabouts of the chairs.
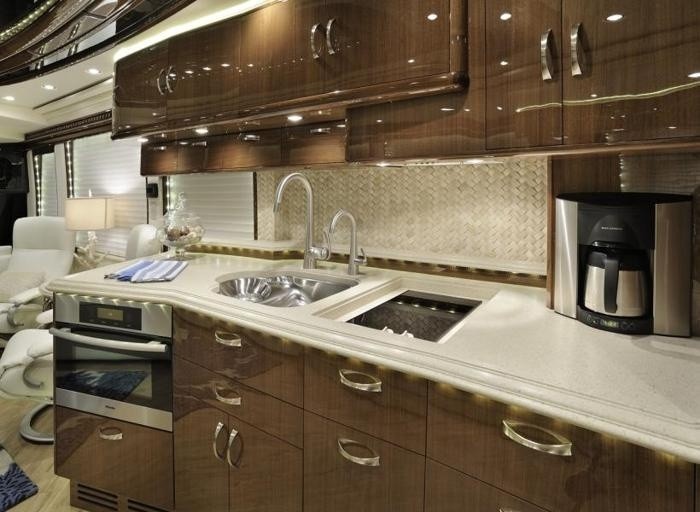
[0,223,164,445]
[0,216,77,350]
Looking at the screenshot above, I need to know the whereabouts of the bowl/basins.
[217,276,272,305]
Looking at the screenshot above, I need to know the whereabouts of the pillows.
[0,263,47,302]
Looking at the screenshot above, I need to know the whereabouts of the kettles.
[581,242,657,332]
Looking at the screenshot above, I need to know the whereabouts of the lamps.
[65,195,117,272]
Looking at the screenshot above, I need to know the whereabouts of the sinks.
[209,268,360,308]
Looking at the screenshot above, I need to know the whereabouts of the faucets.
[329,209,368,276]
[274,172,329,268]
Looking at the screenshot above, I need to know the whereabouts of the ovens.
[50,291,175,435]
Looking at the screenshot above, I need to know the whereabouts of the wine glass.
[156,190,207,261]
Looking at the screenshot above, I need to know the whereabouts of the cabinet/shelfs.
[111,0,700,176]
[52,294,700,512]
